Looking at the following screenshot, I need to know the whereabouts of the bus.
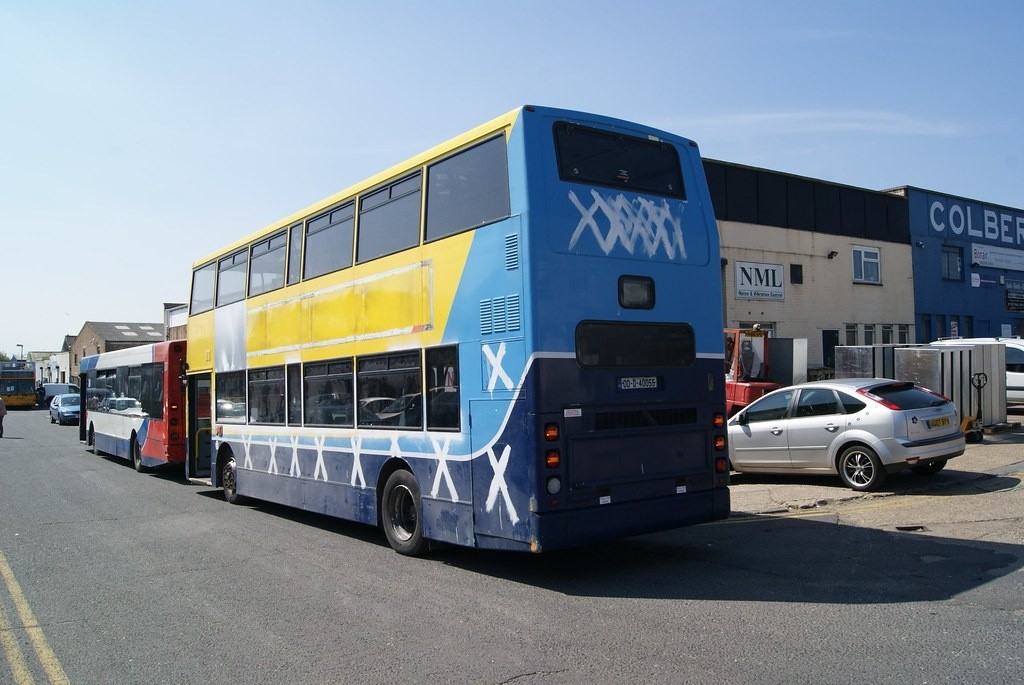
[178,105,732,557]
[79,338,211,478]
[0,370,36,409]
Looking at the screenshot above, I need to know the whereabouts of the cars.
[99,397,149,417]
[725,378,966,494]
[49,393,81,426]
[216,386,461,429]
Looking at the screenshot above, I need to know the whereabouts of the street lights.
[16,344,23,367]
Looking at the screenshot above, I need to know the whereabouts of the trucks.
[43,382,80,408]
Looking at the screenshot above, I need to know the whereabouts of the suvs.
[927,336,1024,411]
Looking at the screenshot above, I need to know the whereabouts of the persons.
[38,383,46,403]
[0,397,7,437]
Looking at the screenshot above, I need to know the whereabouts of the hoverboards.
[960,372,988,443]
